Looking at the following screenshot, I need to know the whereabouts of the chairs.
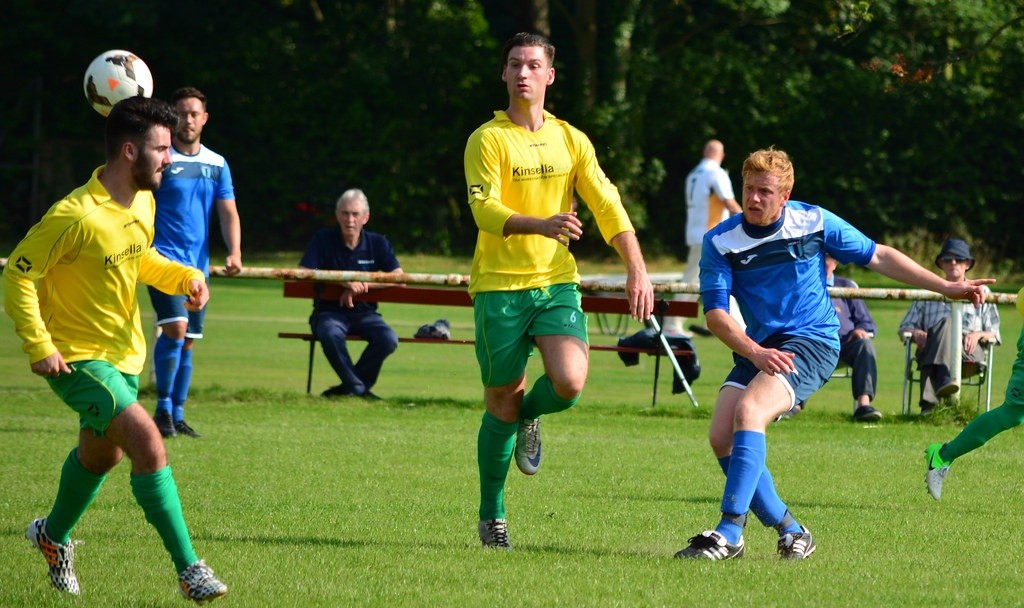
[897,331,994,420]
[830,331,875,416]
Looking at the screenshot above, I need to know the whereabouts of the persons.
[674,142,996,563]
[144,88,241,437]
[684,140,744,335]
[772,244,882,423]
[298,188,403,402]
[924,288,1024,501]
[463,31,655,552]
[896,237,1000,420]
[1,98,229,603]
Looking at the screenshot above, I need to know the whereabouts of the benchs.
[273,280,701,411]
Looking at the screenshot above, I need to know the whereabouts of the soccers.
[83,49,155,118]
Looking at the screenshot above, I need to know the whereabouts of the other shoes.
[853,405,883,424]
[930,365,960,397]
[662,330,694,338]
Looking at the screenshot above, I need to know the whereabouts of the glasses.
[940,255,968,265]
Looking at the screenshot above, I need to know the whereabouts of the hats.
[935,238,975,272]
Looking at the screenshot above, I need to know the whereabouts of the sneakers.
[30,519,81,597]
[776,524,816,561]
[923,442,950,502]
[674,530,745,563]
[176,558,227,600]
[478,519,511,549]
[152,407,177,438]
[175,419,203,439]
[515,418,543,475]
